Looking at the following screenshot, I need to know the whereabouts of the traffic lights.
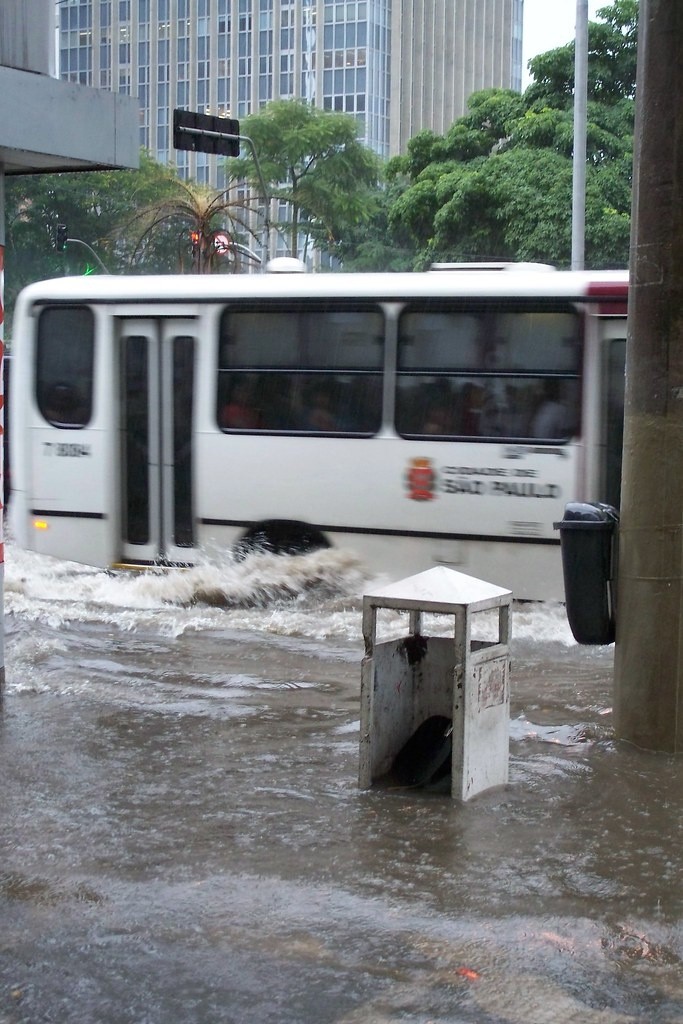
[56,223,71,254]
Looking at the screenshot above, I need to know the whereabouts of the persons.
[40,373,575,438]
[221,375,260,429]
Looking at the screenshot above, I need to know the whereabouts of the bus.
[4,258,631,604]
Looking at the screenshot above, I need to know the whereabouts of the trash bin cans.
[552,500,617,647]
